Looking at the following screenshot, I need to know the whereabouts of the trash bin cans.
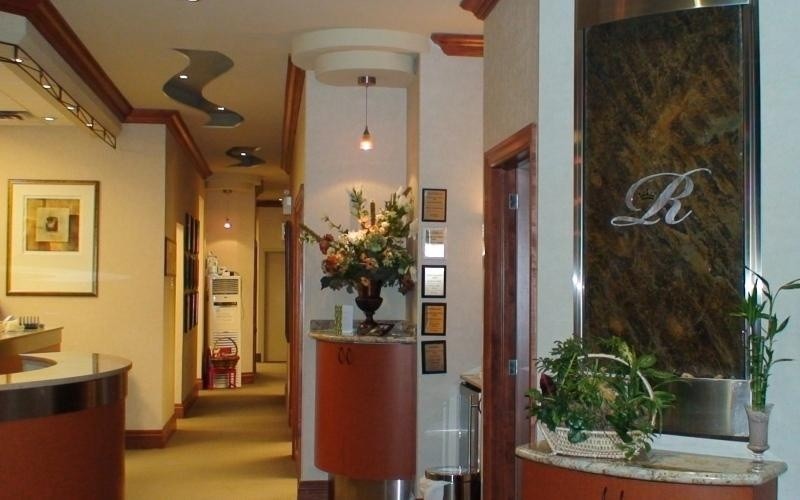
[424,465,480,500]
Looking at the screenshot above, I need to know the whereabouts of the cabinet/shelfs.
[457,381,482,472]
[306,325,417,483]
[515,439,789,499]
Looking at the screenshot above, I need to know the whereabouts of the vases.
[350,282,389,335]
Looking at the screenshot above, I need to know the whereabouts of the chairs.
[204,346,238,390]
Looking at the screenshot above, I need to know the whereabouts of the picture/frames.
[421,185,450,377]
[6,177,103,297]
[163,237,178,278]
[181,212,201,334]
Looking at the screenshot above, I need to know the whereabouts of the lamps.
[355,76,378,152]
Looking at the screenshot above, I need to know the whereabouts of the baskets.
[211,337,240,369]
[536,350,657,463]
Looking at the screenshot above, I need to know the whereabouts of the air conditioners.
[206,270,243,391]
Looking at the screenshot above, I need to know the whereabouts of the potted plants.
[727,265,800,453]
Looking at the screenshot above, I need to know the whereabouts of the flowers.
[298,184,422,293]
